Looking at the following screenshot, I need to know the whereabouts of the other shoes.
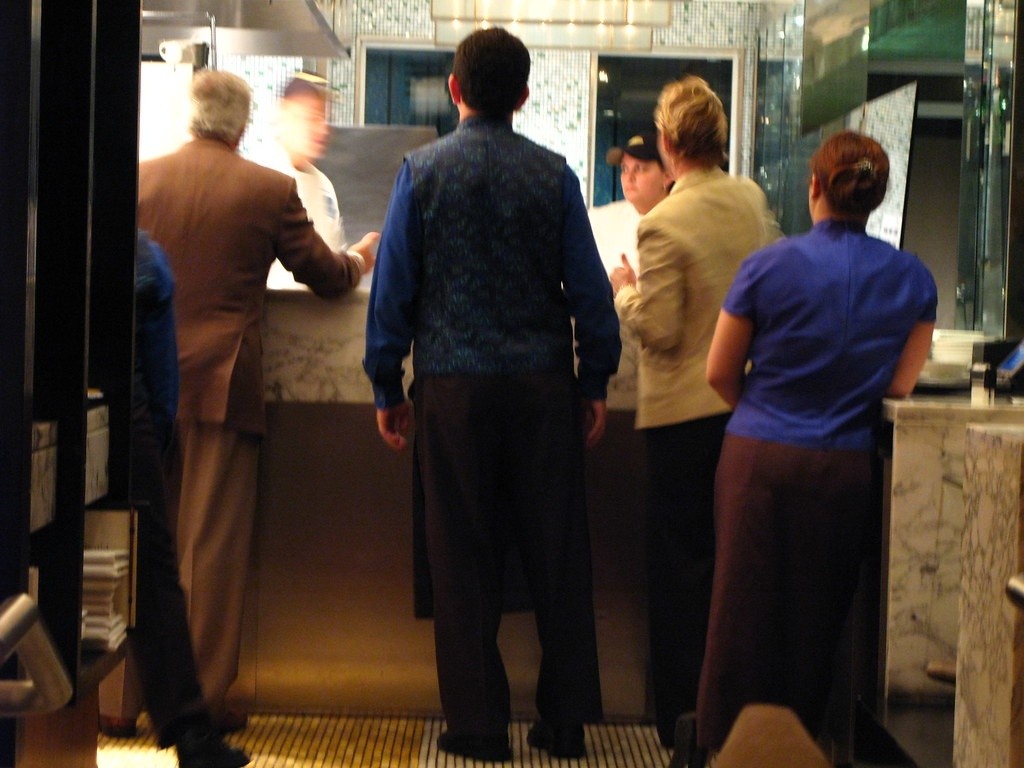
[436,730,512,761]
[206,707,248,730]
[96,712,138,738]
[527,726,586,759]
[179,744,250,768]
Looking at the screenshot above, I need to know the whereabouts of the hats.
[607,128,661,163]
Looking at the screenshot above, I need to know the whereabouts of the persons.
[96,70,380,744]
[242,71,350,286]
[120,223,252,768]
[692,130,941,768]
[364,28,622,761]
[584,131,673,288]
[605,73,787,749]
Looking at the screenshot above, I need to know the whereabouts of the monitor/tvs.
[996,337,1024,378]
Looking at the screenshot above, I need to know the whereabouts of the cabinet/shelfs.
[0,0,145,768]
